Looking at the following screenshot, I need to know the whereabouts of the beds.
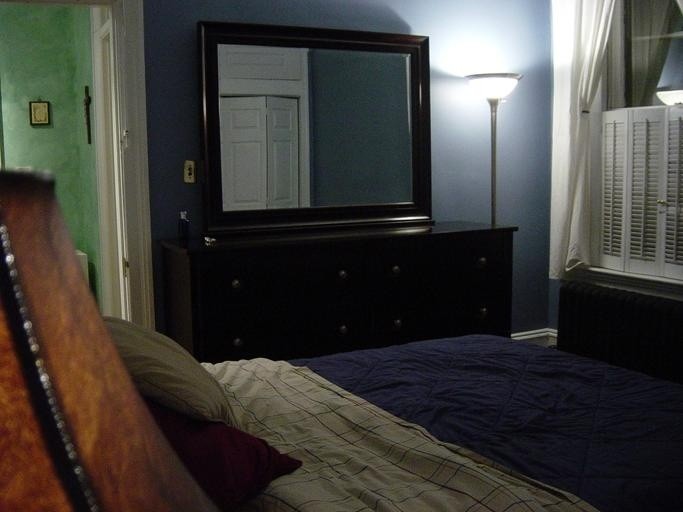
[1,166,681,512]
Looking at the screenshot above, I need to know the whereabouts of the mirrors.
[197,17,437,238]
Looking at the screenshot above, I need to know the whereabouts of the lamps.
[468,73,522,225]
[655,89,682,106]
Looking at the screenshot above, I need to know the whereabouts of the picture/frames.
[29,100,51,126]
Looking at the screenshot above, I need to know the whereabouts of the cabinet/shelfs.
[160,220,519,363]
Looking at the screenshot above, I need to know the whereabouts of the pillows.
[100,314,302,510]
